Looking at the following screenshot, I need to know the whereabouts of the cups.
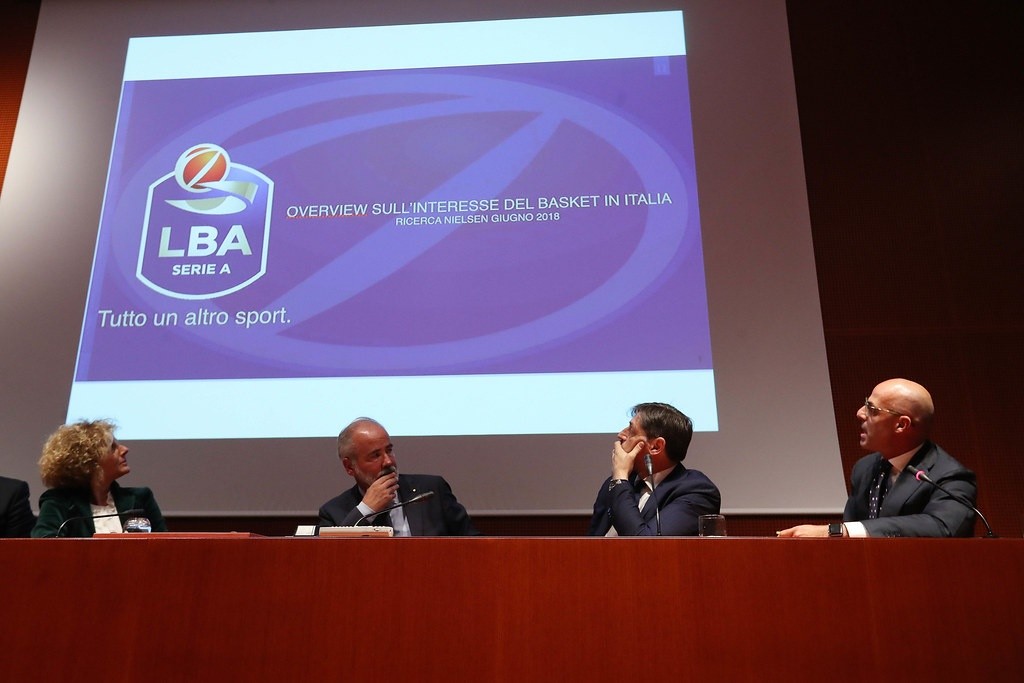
[123,517,151,534]
[698,514,728,536]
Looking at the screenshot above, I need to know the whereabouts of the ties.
[633,480,646,493]
[868,461,893,519]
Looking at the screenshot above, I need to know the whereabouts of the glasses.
[864,397,915,427]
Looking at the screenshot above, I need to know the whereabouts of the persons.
[0,475,34,538]
[589,401,721,537]
[775,377,976,538]
[318,418,482,536]
[30,418,169,539]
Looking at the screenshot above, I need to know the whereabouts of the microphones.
[906,465,996,538]
[354,490,435,527]
[644,453,661,536]
[57,509,145,537]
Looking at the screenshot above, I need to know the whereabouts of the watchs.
[827,522,844,538]
[608,478,627,492]
